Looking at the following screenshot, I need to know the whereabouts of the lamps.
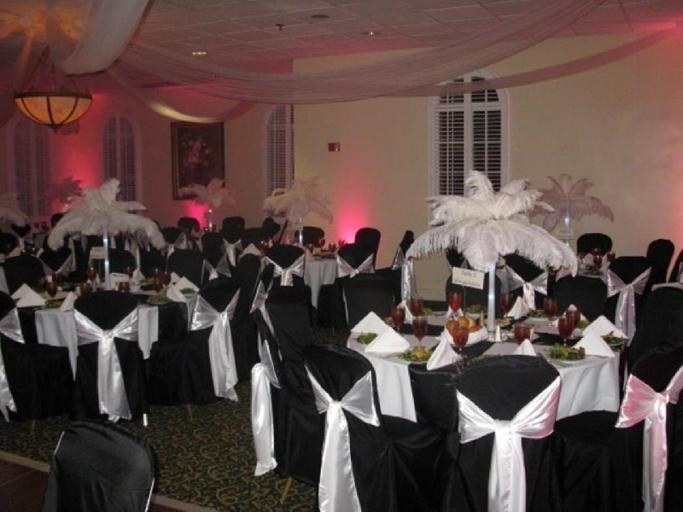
[12,46,93,133]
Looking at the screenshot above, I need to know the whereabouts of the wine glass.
[390,304,407,333]
[452,326,469,354]
[500,292,515,318]
[448,291,463,320]
[558,317,573,348]
[410,316,429,350]
[543,296,558,327]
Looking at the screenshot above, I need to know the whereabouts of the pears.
[446,315,477,336]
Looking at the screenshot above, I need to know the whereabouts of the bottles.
[495,322,502,343]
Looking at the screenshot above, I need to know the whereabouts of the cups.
[410,294,424,316]
[514,324,531,345]
[566,310,581,326]
[258,239,346,255]
[38,266,165,299]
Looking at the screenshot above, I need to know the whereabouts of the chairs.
[1,217,682,510]
